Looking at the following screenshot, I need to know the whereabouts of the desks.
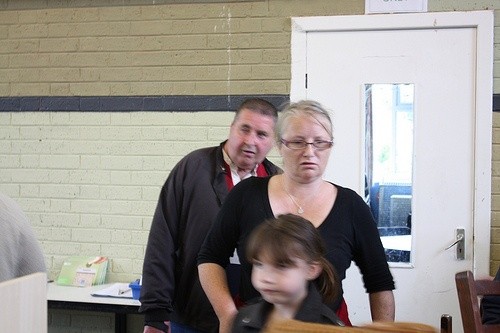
[46,280,144,333]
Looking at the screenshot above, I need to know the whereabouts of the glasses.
[279,137,333,151]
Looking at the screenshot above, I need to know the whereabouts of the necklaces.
[279,179,321,214]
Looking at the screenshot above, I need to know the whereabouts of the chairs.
[0,272,49,333]
[455,270,500,333]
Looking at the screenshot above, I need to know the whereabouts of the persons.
[196,100,396,333]
[232,214,346,333]
[139,97,284,333]
[0,191,47,283]
